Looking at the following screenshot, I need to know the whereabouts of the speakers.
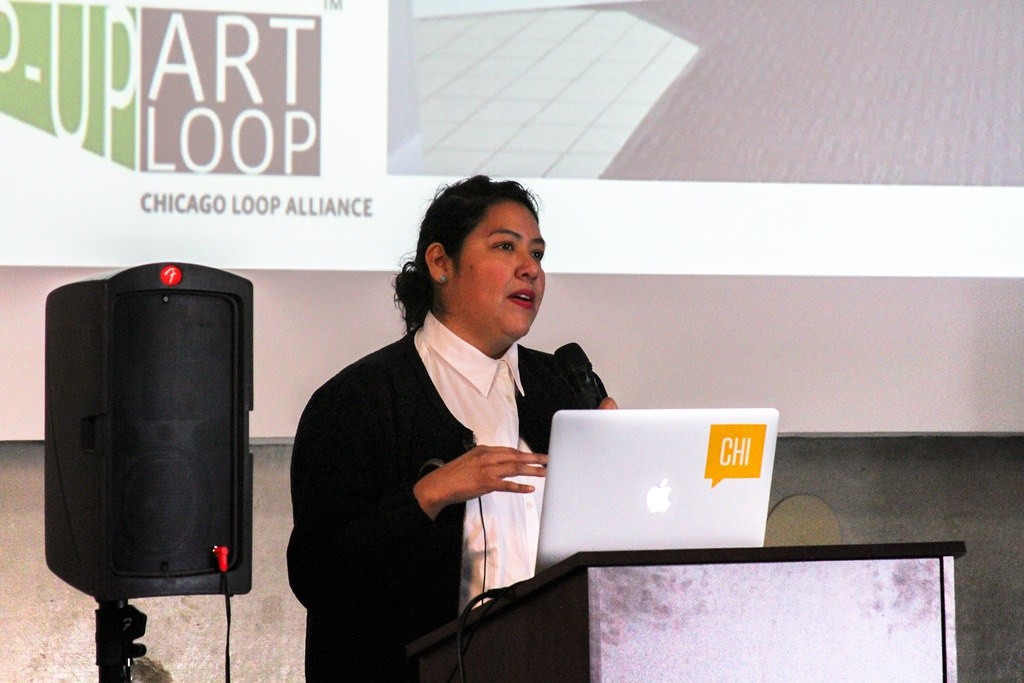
[44,262,255,598]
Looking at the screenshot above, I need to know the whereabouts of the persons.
[287,176,619,683]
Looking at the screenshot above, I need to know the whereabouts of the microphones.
[554,341,606,409]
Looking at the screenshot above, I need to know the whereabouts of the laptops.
[533,408,779,575]
